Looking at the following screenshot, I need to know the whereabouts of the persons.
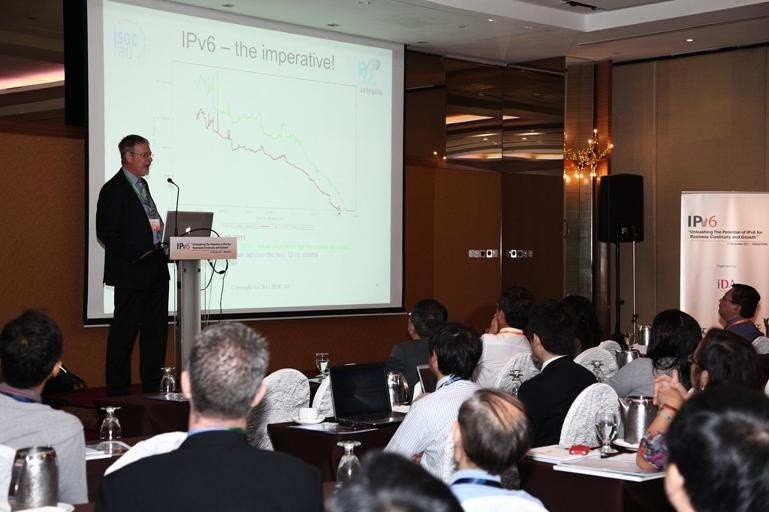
[3,281,769,512]
[95,135,171,399]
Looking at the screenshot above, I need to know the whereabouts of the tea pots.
[618,394,658,444]
[637,322,652,345]
[6,447,60,508]
[386,371,410,413]
[616,349,642,369]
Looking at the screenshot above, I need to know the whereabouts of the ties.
[136,179,162,241]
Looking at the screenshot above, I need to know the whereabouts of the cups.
[297,407,319,420]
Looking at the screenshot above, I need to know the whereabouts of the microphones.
[167,177,180,239]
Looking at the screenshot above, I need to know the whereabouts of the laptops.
[162,211,214,245]
[416,364,436,394]
[328,362,408,425]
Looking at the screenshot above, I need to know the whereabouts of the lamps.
[564,127,613,182]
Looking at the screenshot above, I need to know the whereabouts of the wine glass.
[96,406,124,450]
[159,366,177,395]
[315,353,329,377]
[595,411,618,453]
[336,439,364,491]
[625,331,636,351]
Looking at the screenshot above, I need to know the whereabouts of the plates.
[292,415,324,424]
[524,443,598,462]
[612,439,639,451]
[1,500,76,511]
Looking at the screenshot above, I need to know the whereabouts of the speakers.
[597,174,645,243]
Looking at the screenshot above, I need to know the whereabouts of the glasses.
[722,294,735,305]
[129,151,151,158]
